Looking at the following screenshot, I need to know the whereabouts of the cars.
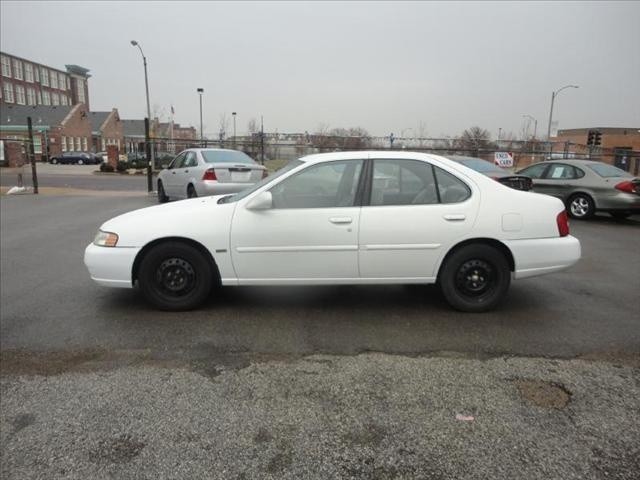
[292,161,424,201]
[513,158,640,218]
[394,156,534,194]
[157,148,270,196]
[83,150,583,315]
[47,149,95,164]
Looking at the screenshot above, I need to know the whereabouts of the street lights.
[232,111,237,148]
[130,39,156,170]
[548,84,579,141]
[521,114,537,139]
[197,87,205,148]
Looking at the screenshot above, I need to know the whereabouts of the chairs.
[327,190,355,206]
[413,183,442,205]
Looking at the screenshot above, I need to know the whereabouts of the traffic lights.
[587,130,595,145]
[595,131,602,146]
